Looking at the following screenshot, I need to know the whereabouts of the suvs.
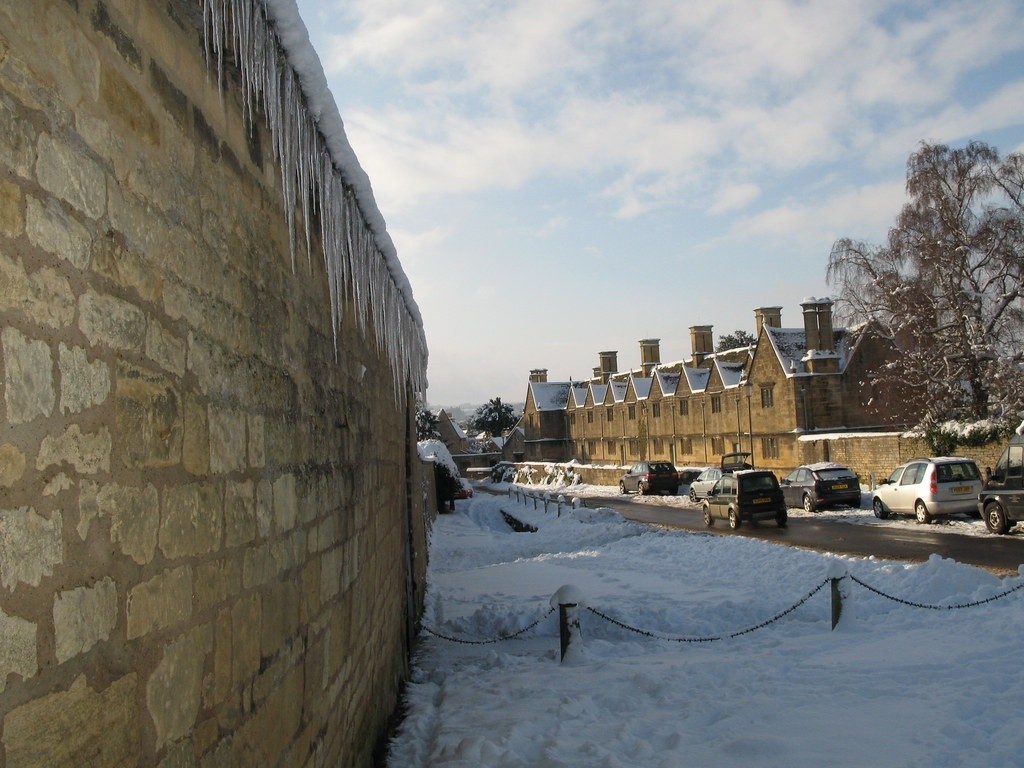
[873,455,984,524]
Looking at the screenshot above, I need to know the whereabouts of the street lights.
[744,381,754,470]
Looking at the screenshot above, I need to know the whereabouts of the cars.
[702,470,787,530]
[688,452,753,503]
[453,478,473,499]
[779,461,862,513]
[619,461,679,496]
[977,433,1024,535]
